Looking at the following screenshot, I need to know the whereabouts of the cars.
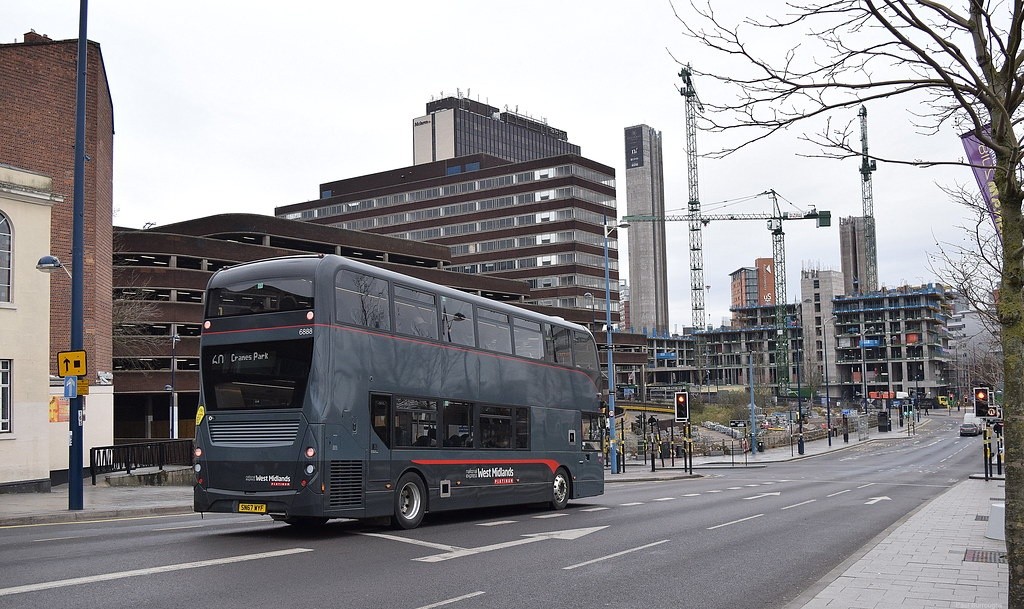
[959,423,981,436]
[891,397,916,410]
[802,409,819,424]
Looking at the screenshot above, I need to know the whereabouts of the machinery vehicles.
[857,105,909,408]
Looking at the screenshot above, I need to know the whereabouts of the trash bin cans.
[659,441,670,458]
[877,411,888,432]
[676,445,682,458]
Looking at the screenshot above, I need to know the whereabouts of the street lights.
[853,327,876,415]
[795,298,812,455]
[602,207,631,475]
[443,304,465,343]
[164,336,181,438]
[584,292,596,440]
[37,0,89,510]
[885,336,897,417]
[973,340,982,386]
[823,316,837,446]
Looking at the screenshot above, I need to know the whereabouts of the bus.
[192,253,604,531]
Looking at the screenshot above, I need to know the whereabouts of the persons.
[924,405,930,416]
[957,400,960,411]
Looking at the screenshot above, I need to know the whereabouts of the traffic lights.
[973,387,989,417]
[903,405,908,416]
[989,392,995,406]
[675,392,690,420]
[635,413,642,430]
[908,404,913,415]
[965,397,968,403]
[949,394,953,402]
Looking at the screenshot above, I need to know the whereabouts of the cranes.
[620,188,831,397]
[677,52,710,385]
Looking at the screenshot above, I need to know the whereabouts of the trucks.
[915,393,949,409]
[869,391,908,405]
[963,413,982,433]
